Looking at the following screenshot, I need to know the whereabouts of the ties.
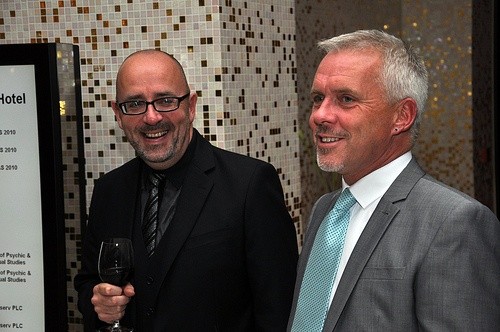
[291,187,358,332]
[148,172,166,261]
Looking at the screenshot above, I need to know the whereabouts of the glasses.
[115,92,190,115]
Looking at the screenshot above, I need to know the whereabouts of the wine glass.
[98,238,135,332]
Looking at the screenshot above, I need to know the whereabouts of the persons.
[284,29,500,332]
[73,50,300,332]
